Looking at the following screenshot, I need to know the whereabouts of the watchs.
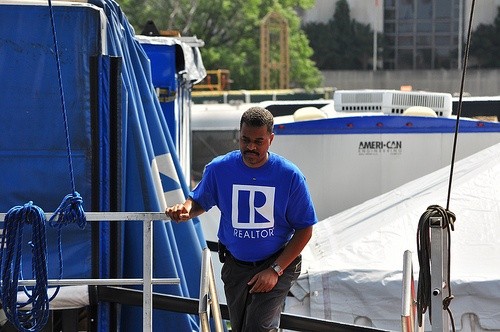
[270,262,283,277]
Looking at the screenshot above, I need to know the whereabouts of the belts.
[232,256,272,267]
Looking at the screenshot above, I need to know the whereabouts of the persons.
[166,106,318,332]
